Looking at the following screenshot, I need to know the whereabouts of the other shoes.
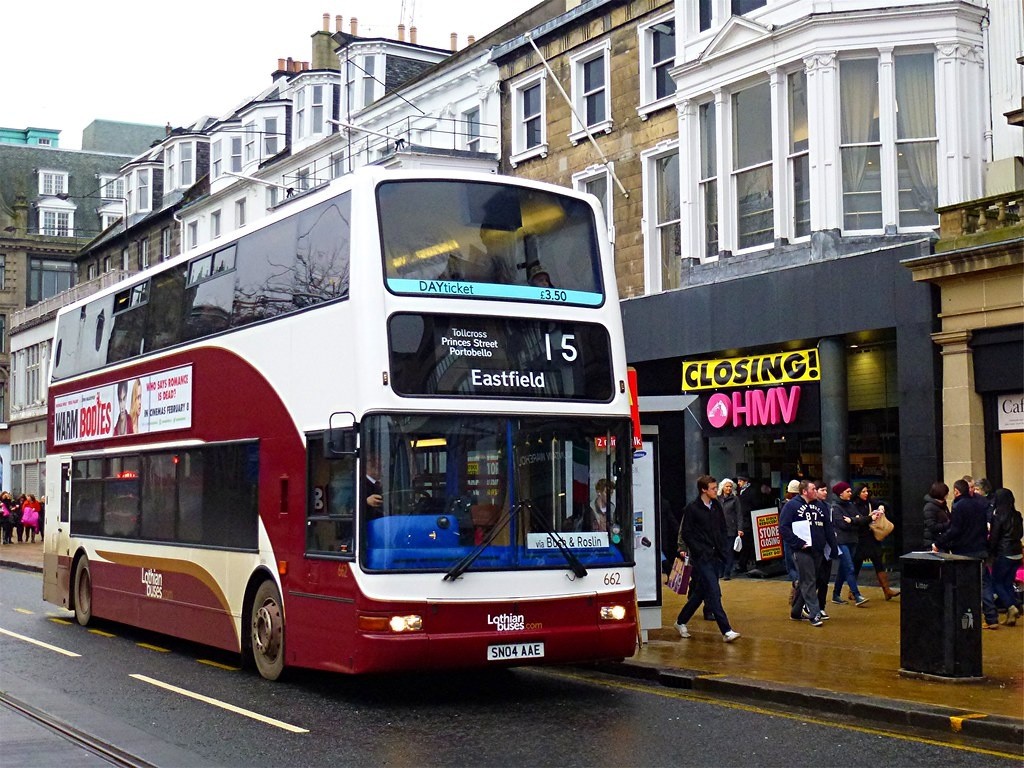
[855,597,870,606]
[831,596,848,605]
[705,612,716,622]
[790,613,808,620]
[982,622,998,628]
[723,574,731,579]
[821,610,830,620]
[810,616,823,626]
[3,539,44,544]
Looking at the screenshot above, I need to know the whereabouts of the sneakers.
[673,619,692,639]
[722,631,740,643]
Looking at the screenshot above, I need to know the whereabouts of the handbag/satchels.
[868,509,894,540]
[22,507,38,527]
[667,555,691,595]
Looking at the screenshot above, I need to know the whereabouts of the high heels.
[1001,604,1020,625]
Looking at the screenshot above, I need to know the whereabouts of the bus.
[41,166,640,683]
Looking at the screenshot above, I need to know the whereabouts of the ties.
[374,481,382,489]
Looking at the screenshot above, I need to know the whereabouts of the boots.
[849,574,860,599]
[877,571,901,600]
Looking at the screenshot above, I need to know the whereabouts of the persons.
[351,446,401,542]
[13,494,27,542]
[716,478,743,581]
[932,476,999,629]
[779,479,829,626]
[757,479,774,508]
[783,479,837,619]
[38,494,44,542]
[738,475,757,575]
[576,479,618,533]
[832,483,870,606]
[129,378,141,436]
[848,482,900,600]
[922,482,951,555]
[0,491,15,545]
[526,266,553,288]
[22,494,41,543]
[987,490,1024,626]
[113,381,134,436]
[673,475,742,642]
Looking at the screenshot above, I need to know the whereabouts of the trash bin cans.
[900,552,983,678]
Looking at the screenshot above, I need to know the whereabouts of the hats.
[788,480,801,493]
[833,481,851,497]
